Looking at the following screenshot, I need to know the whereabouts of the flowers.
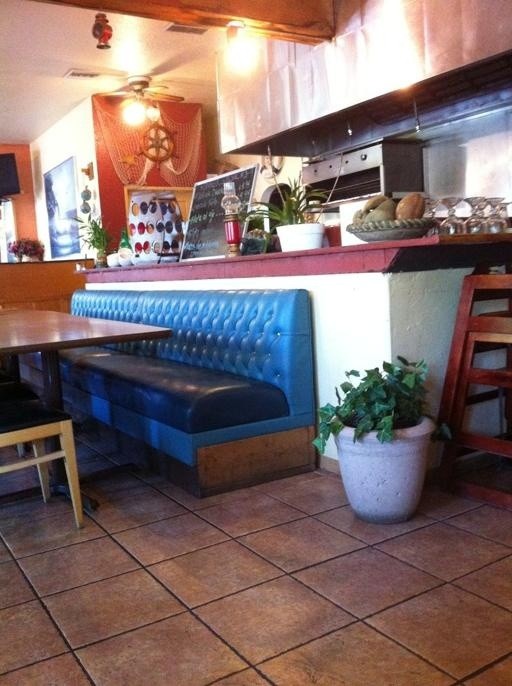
[7,237,45,263]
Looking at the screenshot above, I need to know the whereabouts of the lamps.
[91,9,113,50]
[119,75,162,126]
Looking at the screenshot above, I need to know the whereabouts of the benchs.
[19,290,317,498]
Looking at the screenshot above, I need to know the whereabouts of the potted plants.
[312,355,452,524]
[241,172,329,253]
[72,213,115,268]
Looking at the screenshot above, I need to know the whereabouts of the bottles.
[119,227,132,250]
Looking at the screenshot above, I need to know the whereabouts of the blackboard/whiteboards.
[179,164,259,263]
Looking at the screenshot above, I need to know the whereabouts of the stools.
[1,397,85,529]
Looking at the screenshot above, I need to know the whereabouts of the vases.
[22,254,39,263]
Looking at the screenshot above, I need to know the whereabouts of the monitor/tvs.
[0,152,22,197]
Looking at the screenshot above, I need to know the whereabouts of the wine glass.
[423,196,512,238]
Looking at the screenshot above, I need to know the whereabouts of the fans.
[96,76,188,102]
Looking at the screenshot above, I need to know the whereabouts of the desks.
[1,309,172,510]
